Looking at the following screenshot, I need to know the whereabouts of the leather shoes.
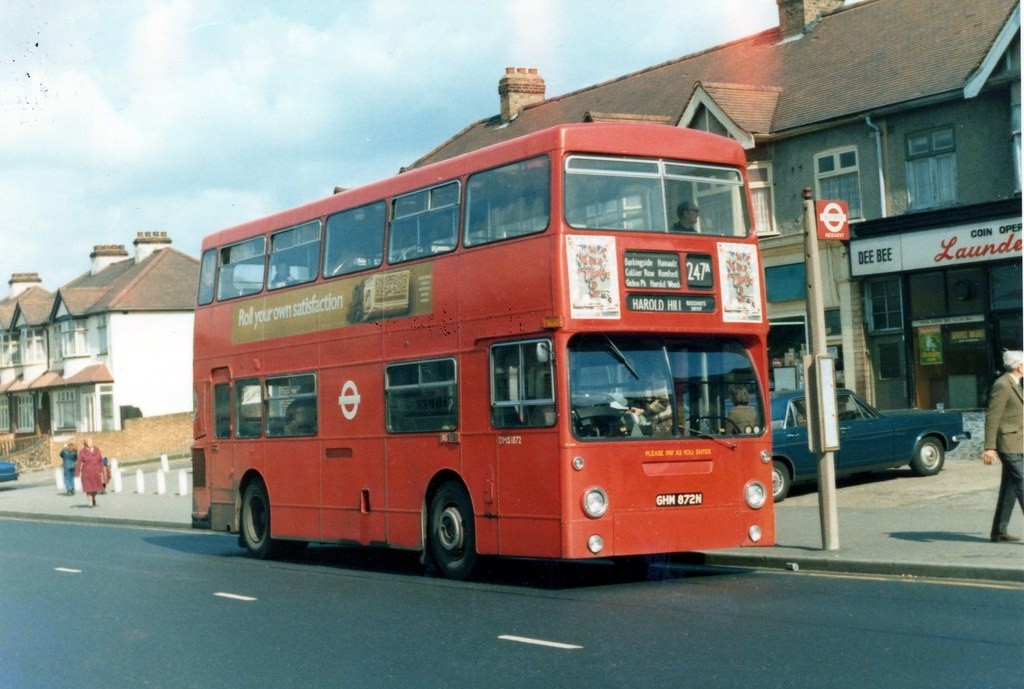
[993,532,1020,542]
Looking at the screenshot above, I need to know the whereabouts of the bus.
[0,462,20,484]
[187,119,778,579]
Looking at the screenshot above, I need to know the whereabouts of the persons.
[282,406,315,435]
[60,440,77,496]
[669,201,700,232]
[75,437,105,506]
[102,457,110,494]
[270,259,296,289]
[724,385,757,433]
[982,348,1024,542]
[629,385,673,436]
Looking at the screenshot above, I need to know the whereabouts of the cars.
[763,384,969,503]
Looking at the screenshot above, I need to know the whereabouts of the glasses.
[689,209,700,213]
